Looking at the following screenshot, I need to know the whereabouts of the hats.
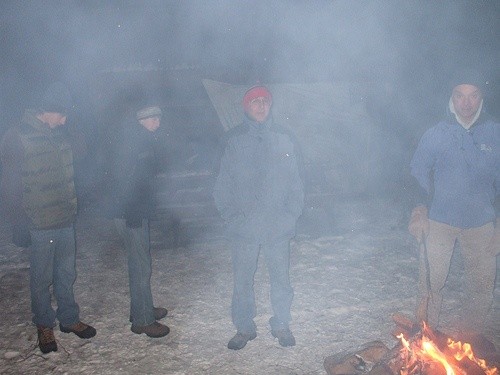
[136,96,162,119]
[243,80,272,107]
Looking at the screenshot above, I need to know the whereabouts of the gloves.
[127,213,142,228]
[12,231,32,247]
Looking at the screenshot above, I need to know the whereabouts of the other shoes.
[270,327,295,347]
[131,322,170,338]
[130,308,167,321]
[37,327,58,353]
[61,320,96,338]
[228,332,257,350]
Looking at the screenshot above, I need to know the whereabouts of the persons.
[410,70,500,353]
[0,81,96,353]
[213,79,309,349]
[105,103,170,337]
